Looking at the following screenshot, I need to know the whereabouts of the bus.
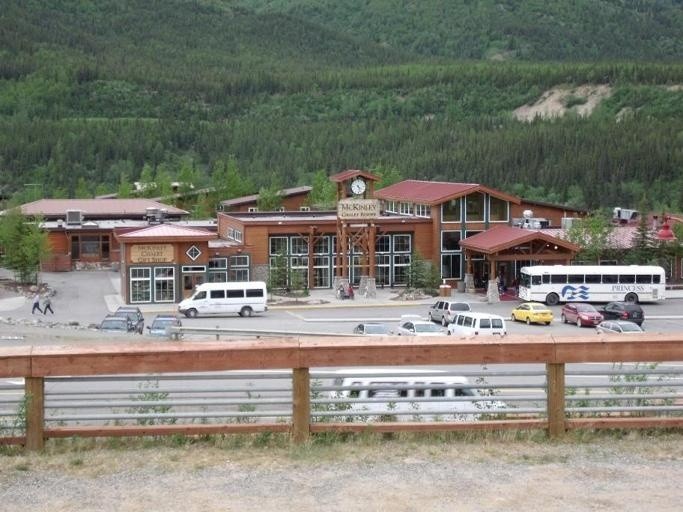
[514,261,667,308]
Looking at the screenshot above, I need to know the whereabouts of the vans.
[442,312,509,339]
[328,370,516,421]
[176,279,269,319]
[427,299,471,327]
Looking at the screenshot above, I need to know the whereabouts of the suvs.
[146,315,185,341]
[113,305,145,334]
[97,313,136,335]
[595,300,646,328]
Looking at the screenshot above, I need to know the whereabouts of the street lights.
[653,215,683,243]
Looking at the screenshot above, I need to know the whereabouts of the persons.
[29,291,42,315]
[480,270,520,297]
[42,295,54,314]
[347,286,353,299]
[337,285,344,299]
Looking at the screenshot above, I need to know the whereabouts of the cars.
[560,301,604,328]
[591,318,646,336]
[509,302,554,326]
[352,321,392,336]
[395,320,444,335]
